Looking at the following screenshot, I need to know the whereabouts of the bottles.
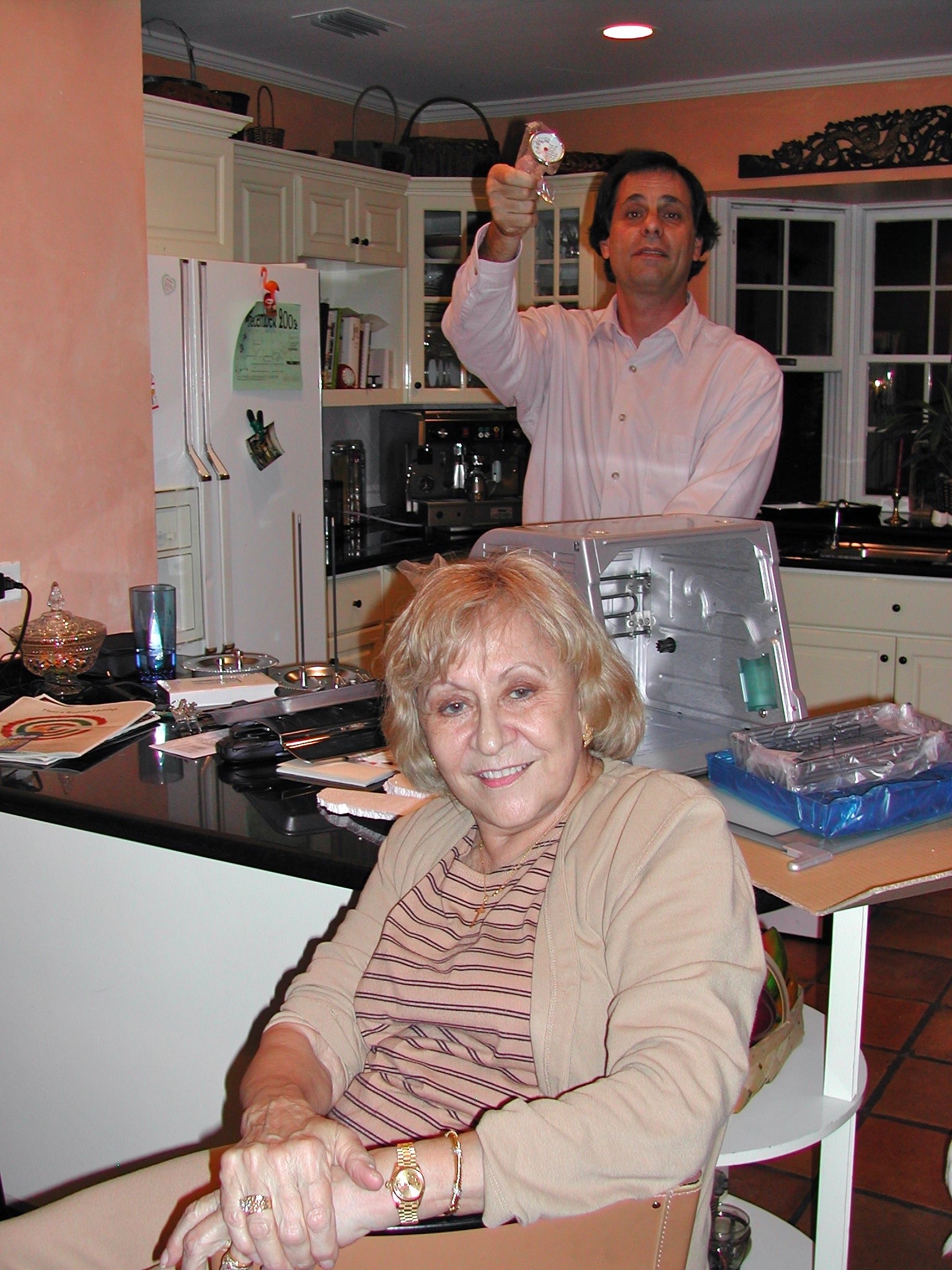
[329,438,369,525]
[11,580,109,696]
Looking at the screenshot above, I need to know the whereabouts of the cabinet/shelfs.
[758,571,951,939]
[715,905,872,1270]
[328,555,464,683]
[294,152,408,267]
[405,171,615,411]
[142,93,255,262]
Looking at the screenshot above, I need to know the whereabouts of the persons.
[441,120,782,526]
[159,545,767,1270]
[261,445,277,465]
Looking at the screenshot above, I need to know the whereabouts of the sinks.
[836,542,951,563]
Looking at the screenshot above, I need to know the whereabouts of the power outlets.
[0,561,21,604]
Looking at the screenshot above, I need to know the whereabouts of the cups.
[129,583,178,688]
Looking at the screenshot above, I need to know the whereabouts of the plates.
[181,654,278,673]
[271,664,372,691]
[424,285,445,320]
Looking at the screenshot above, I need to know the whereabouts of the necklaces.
[469,755,595,927]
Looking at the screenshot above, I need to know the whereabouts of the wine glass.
[425,329,476,388]
[542,218,579,258]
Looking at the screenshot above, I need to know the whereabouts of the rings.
[222,1250,253,1270]
[222,1240,231,1251]
[240,1194,272,1214]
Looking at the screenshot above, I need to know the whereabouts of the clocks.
[337,364,356,389]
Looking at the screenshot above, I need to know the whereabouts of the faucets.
[830,499,851,551]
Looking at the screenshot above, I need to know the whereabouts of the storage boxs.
[156,673,279,710]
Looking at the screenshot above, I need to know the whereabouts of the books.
[0,694,161,765]
[276,758,401,792]
[319,302,394,390]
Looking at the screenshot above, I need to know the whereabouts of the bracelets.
[443,1131,463,1215]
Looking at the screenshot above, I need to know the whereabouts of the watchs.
[386,1142,425,1225]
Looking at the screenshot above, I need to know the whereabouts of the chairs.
[206,1116,736,1270]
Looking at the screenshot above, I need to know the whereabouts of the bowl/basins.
[426,247,461,259]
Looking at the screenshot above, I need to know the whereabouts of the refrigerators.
[147,256,329,663]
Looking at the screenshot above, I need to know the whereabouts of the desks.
[231,139,295,263]
